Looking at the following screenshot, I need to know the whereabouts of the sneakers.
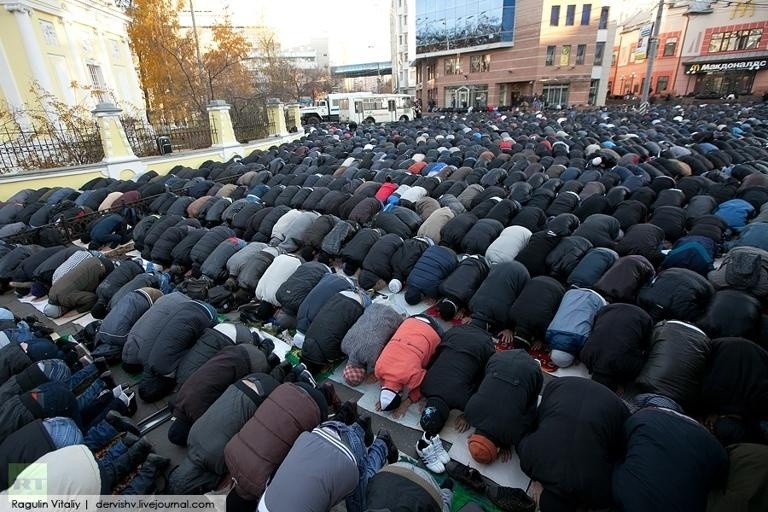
[25,314,171,466]
[251,328,528,511]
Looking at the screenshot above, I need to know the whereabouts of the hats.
[324,352,499,461]
[301,247,571,368]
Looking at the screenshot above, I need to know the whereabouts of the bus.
[340,93,416,125]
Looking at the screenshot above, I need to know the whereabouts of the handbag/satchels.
[185,276,236,314]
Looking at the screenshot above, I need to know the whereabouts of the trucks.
[299,92,373,124]
